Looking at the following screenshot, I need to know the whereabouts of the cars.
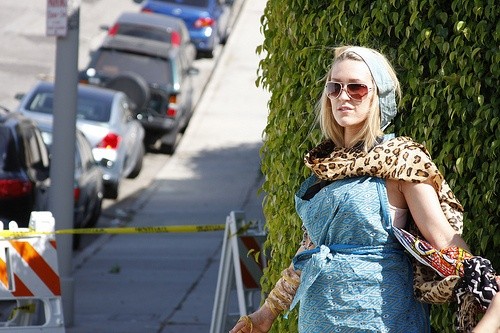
[0,105,103,250]
[133,0,225,58]
[14,81,146,199]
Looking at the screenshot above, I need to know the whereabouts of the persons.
[229,46,500,333]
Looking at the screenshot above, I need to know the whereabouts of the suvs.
[78,11,199,131]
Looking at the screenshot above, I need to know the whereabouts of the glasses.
[325,81,376,101]
